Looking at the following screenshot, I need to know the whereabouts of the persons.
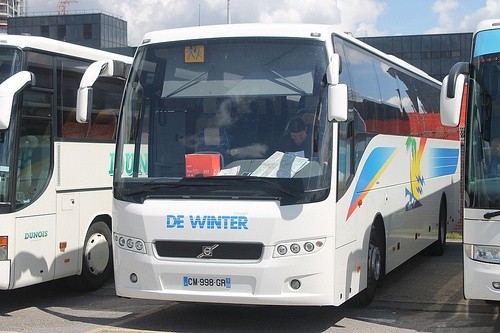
[278,119,313,153]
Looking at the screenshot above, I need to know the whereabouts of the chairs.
[194,95,320,167]
[22,107,117,141]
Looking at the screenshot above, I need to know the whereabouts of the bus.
[0,34,246,294]
[438,18,500,308]
[75,23,462,311]
